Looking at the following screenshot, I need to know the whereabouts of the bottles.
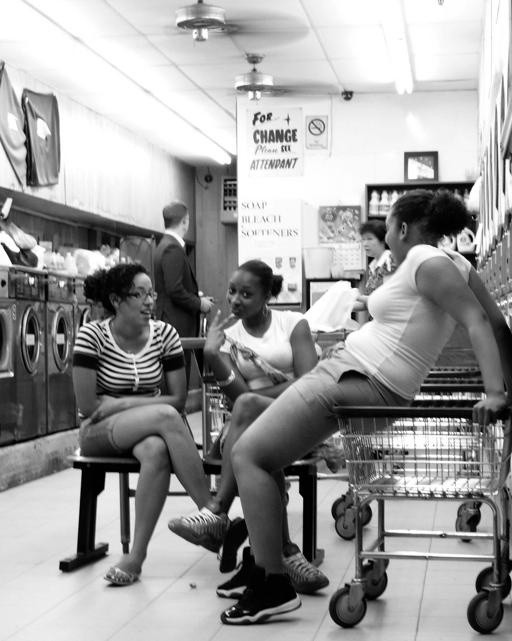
[64,251,78,273]
[366,188,470,217]
[45,249,67,269]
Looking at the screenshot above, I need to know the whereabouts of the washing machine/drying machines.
[16,266,51,439]
[49,270,80,433]
[0,262,18,446]
[76,274,96,426]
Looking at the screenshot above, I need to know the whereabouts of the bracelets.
[485,388,505,396]
[217,370,236,387]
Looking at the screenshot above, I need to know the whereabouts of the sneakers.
[102,505,329,623]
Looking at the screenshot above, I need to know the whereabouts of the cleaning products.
[367,190,389,217]
[392,189,401,203]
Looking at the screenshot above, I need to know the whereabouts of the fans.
[113,1,310,51]
[173,56,339,102]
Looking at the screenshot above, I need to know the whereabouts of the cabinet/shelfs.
[364,181,477,280]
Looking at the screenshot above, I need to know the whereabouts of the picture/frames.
[404,150,438,181]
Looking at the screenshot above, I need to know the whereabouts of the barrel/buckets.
[439,234,455,250]
[302,244,332,280]
[458,228,474,252]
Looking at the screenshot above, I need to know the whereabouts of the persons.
[168,260,318,553]
[215,189,512,625]
[352,220,398,320]
[72,262,247,586]
[154,202,215,450]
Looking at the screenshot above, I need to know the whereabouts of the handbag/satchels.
[323,432,346,472]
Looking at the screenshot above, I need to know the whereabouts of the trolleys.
[195,338,401,515]
[328,375,510,635]
[331,336,488,541]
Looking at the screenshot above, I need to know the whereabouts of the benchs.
[59,333,330,572]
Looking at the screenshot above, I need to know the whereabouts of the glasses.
[127,290,158,302]
